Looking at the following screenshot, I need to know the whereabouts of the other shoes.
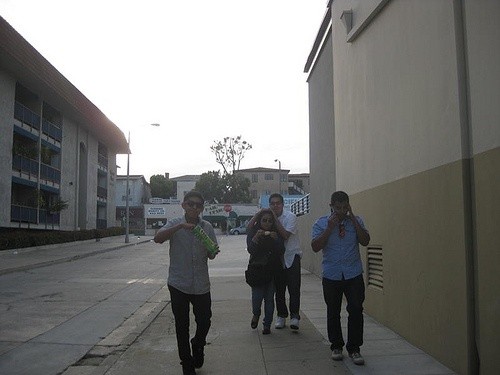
[183,367,196,375]
[191,339,204,368]
[262,323,271,334]
[251,316,259,328]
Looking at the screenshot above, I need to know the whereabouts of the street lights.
[125,123,161,243]
[275,159,281,194]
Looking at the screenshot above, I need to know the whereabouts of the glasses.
[262,218,273,224]
[339,223,345,238]
[186,200,203,209]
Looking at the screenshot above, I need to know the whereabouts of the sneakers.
[275,317,286,328]
[290,318,299,329]
[349,352,364,364]
[332,349,343,360]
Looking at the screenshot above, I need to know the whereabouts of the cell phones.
[330,208,335,216]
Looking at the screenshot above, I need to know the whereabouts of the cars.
[229,223,248,235]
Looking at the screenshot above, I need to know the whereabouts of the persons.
[247,210,283,335]
[248,194,303,330]
[312,191,370,364]
[155,191,220,375]
